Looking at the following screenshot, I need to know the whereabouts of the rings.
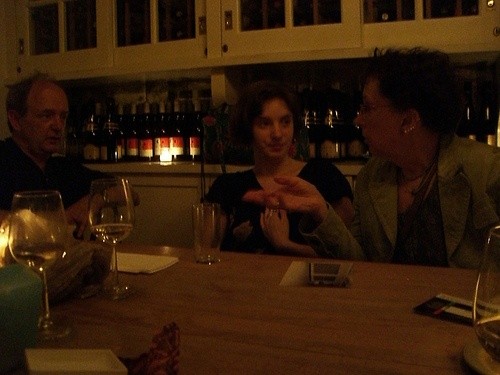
[270,212,277,217]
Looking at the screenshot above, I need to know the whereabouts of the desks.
[0,241,500,375]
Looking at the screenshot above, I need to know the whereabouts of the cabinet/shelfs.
[0,0,500,86]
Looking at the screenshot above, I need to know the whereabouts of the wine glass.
[8,190,72,341]
[88,178,136,301]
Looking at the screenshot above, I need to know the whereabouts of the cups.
[473,225,500,364]
[192,202,220,263]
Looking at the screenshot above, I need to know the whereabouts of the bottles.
[459,79,500,146]
[81,112,100,162]
[101,95,209,161]
[302,82,363,142]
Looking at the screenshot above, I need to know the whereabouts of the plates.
[463,338,500,375]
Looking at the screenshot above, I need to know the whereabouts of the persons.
[200,78,354,259]
[242,44,500,275]
[0,70,140,242]
